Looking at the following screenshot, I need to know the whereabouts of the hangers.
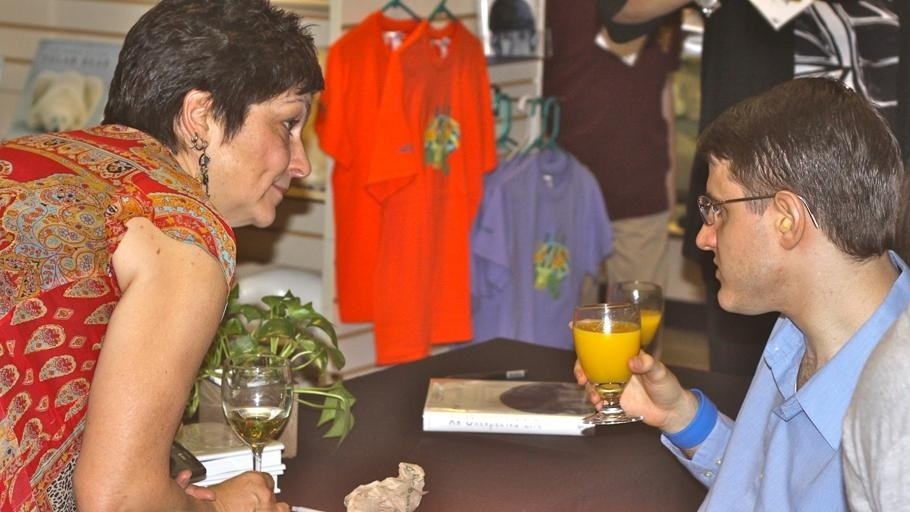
[476,92,596,199]
[331,0,482,61]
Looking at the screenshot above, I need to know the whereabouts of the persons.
[567,75,910,512]
[1,0,326,512]
[483,2,542,61]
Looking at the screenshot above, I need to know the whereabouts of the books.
[189,471,287,496]
[421,372,597,438]
[175,418,287,483]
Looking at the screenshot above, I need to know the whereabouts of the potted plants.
[182,288,355,461]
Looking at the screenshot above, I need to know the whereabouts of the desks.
[168,337,754,511]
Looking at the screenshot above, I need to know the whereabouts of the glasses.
[697,190,777,227]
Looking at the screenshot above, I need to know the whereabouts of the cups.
[612,280,664,352]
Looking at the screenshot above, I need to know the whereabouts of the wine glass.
[570,304,643,426]
[221,354,293,471]
